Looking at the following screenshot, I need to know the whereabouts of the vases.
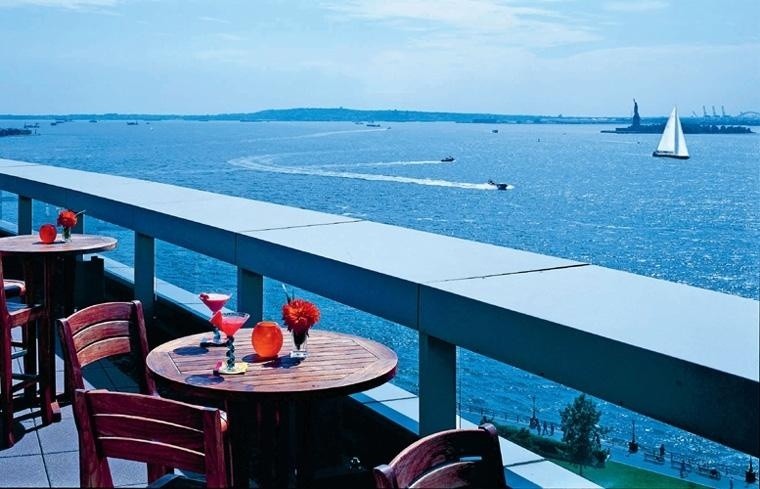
[290,325,307,356]
[62,226,71,242]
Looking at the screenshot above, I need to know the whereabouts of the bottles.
[251,321,283,359]
[39,224,57,244]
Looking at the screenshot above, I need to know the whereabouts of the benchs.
[644,452,722,481]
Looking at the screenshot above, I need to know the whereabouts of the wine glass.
[207,310,250,373]
[197,289,234,345]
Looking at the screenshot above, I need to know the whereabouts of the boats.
[441,156,454,162]
[22,114,141,129]
[351,119,393,131]
[488,179,508,189]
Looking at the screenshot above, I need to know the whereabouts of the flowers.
[283,300,319,329]
[56,212,76,225]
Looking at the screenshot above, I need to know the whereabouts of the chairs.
[58,299,173,483]
[0,260,50,446]
[373,420,506,488]
[75,387,226,488]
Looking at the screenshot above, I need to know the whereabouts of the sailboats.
[654,106,691,159]
